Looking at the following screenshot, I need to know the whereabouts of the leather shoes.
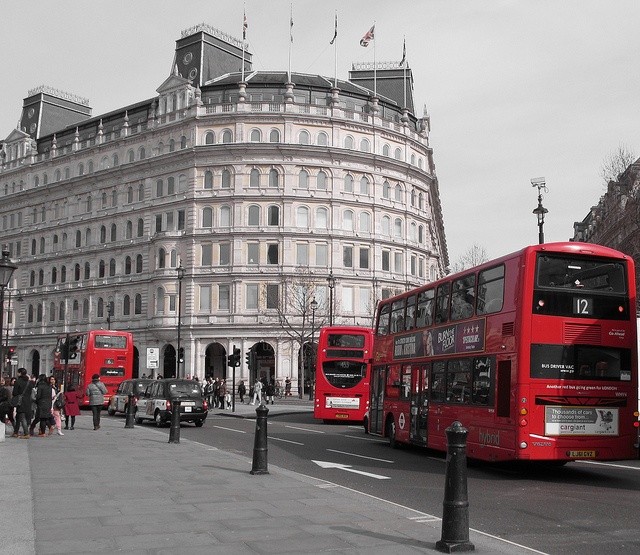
[11,433,18,437]
[18,435,30,439]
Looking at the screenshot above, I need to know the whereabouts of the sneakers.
[219,406,224,408]
[257,402,261,405]
[70,427,74,430]
[39,433,46,437]
[250,402,255,404]
[94,425,100,430]
[48,426,54,435]
[58,430,64,435]
[241,398,243,402]
[64,427,68,429]
[226,407,229,410]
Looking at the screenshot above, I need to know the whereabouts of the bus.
[364,242,640,469]
[49,330,133,407]
[313,327,374,423]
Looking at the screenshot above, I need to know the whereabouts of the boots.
[272,400,274,404]
[267,400,269,404]
[30,423,36,434]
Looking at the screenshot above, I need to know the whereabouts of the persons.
[86,374,108,430]
[63,385,82,429]
[238,381,246,403]
[248,378,275,405]
[143,372,235,412]
[0,367,65,439]
[284,377,292,395]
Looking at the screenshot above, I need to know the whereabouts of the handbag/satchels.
[9,393,23,407]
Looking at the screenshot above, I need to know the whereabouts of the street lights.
[534,193,549,242]
[310,297,317,400]
[0,244,18,382]
[106,302,111,329]
[325,271,336,326]
[4,288,24,354]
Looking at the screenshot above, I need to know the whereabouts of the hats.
[220,378,225,381]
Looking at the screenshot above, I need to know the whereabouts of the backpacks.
[52,385,65,409]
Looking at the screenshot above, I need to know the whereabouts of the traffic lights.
[227,355,233,367]
[57,337,68,360]
[178,348,184,364]
[8,347,14,356]
[233,348,241,366]
[69,335,79,359]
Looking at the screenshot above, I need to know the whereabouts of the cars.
[134,378,208,427]
[108,379,156,415]
